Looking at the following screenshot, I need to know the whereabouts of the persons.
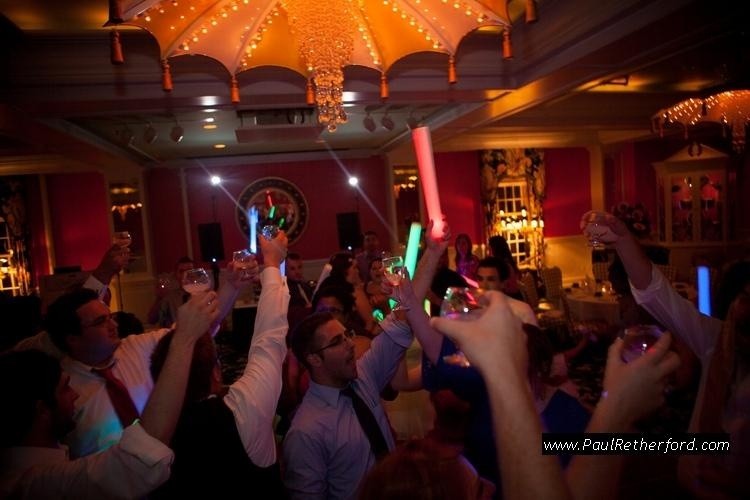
[2,174,750,500]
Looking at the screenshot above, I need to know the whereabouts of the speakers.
[336,211,361,250]
[198,222,225,262]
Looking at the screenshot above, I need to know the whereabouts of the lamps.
[119,121,185,147]
[93,0,537,131]
[650,90,750,153]
[363,111,417,131]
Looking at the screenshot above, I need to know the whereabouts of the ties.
[91,364,139,429]
[340,385,391,462]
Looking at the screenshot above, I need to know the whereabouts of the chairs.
[520,271,566,336]
[542,266,562,300]
[593,262,612,281]
[655,264,676,284]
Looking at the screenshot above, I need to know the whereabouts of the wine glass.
[586,213,609,252]
[382,255,411,311]
[442,287,484,368]
[234,249,256,281]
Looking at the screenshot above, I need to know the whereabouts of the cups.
[181,268,210,294]
[113,230,132,246]
[622,325,662,364]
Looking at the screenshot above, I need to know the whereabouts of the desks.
[562,281,697,337]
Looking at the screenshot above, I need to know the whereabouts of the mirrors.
[236,177,310,249]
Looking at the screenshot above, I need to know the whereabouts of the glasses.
[318,307,343,313]
[319,329,354,350]
[90,314,112,324]
